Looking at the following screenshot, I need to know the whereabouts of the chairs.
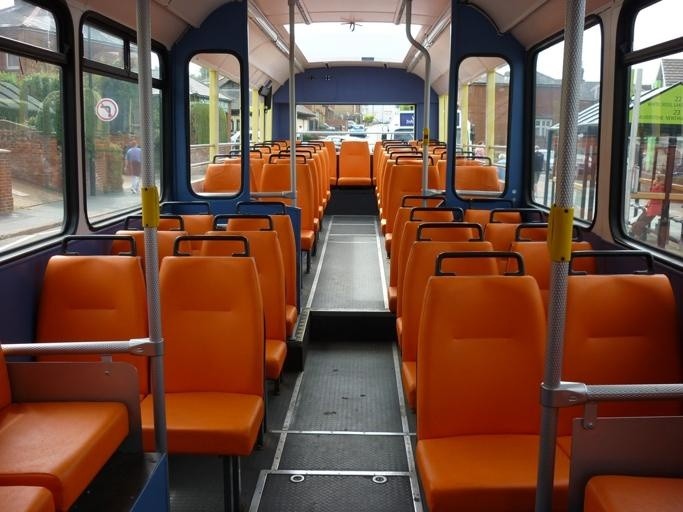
[555,249,677,459]
[210,214,288,394]
[133,233,268,510]
[36,235,150,405]
[0,483,56,511]
[396,206,500,352]
[0,346,132,509]
[581,474,682,511]
[335,139,371,189]
[202,138,337,275]
[108,216,193,283]
[369,136,596,312]
[233,200,298,335]
[413,252,574,511]
[157,201,213,234]
[401,222,501,411]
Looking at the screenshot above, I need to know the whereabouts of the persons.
[123,140,143,194]
[494,154,506,182]
[631,173,667,242]
[229,128,260,155]
[474,140,485,161]
[532,145,544,185]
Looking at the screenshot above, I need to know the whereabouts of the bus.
[380,109,475,161]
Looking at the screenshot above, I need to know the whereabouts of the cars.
[535,149,591,175]
[349,124,367,137]
[347,120,355,128]
[322,135,346,152]
